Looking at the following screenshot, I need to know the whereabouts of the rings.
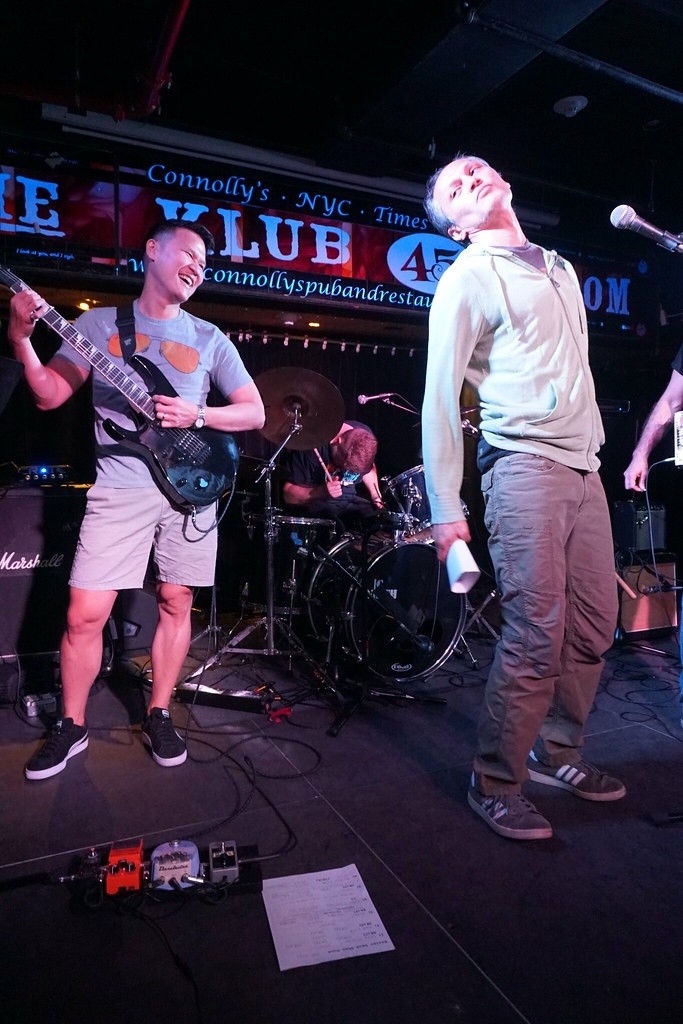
[30,313,38,321]
[163,413,165,419]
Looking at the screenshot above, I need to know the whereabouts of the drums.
[239,510,339,618]
[306,534,471,685]
[379,463,473,541]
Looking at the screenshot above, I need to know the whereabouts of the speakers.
[613,501,666,551]
[614,551,680,640]
[596,417,639,523]
[0,482,161,663]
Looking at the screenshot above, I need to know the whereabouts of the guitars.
[0,266,240,515]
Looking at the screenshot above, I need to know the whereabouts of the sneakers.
[24,718,89,780]
[142,708,187,767]
[528,751,626,801]
[467,771,552,839]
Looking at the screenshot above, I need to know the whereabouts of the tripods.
[604,543,681,662]
[172,402,449,736]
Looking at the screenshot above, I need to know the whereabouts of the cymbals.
[239,453,295,477]
[253,366,345,451]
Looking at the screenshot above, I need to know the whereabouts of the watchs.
[192,403,206,429]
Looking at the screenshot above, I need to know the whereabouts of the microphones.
[610,204,683,254]
[358,393,394,404]
[642,586,683,594]
[412,635,435,656]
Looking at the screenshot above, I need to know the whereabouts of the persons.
[625,344,683,492]
[420,154,628,839]
[9,216,265,780]
[279,420,384,536]
